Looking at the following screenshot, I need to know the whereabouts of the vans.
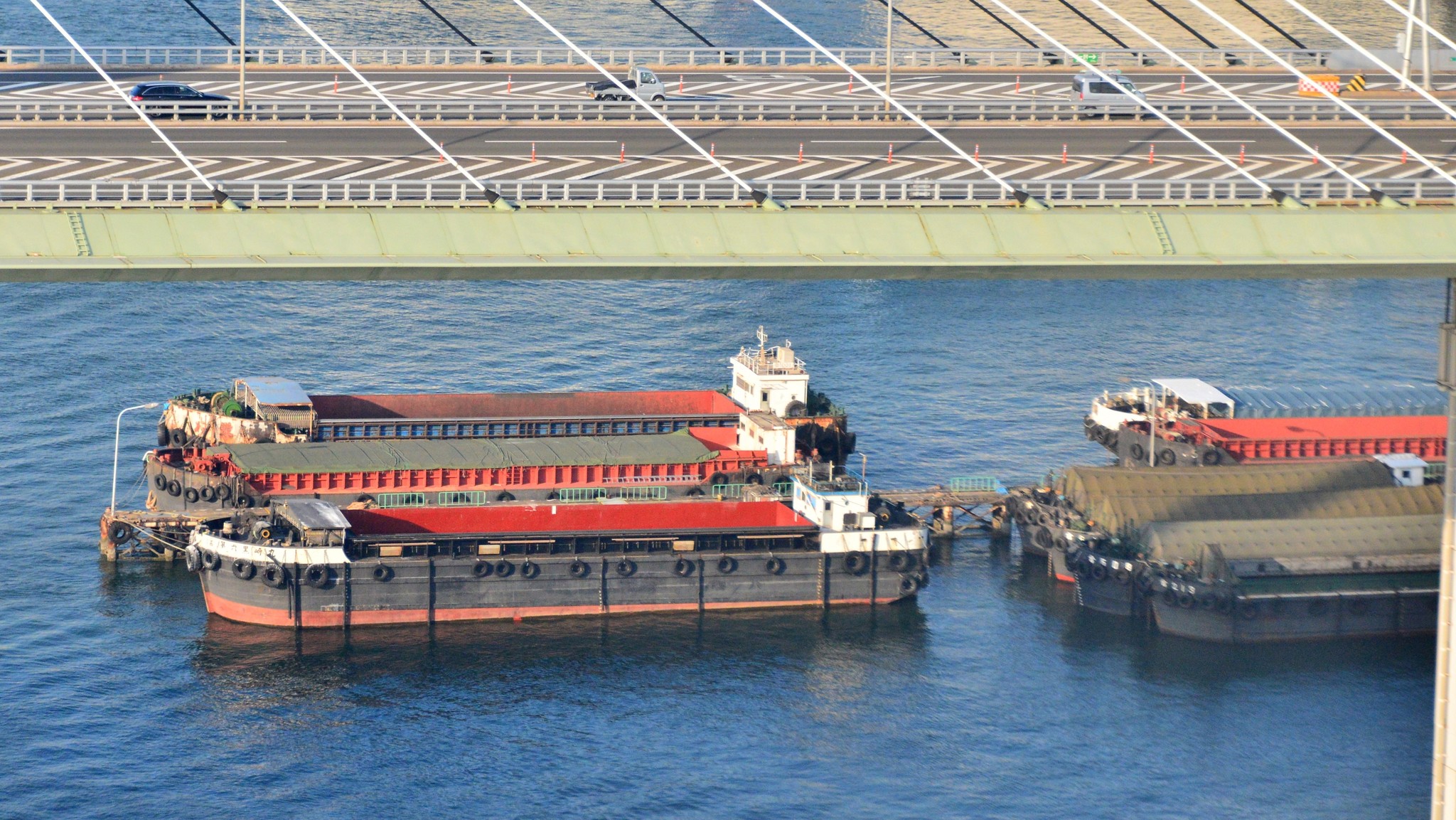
[1070,73,1147,119]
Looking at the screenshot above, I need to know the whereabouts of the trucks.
[585,67,666,108]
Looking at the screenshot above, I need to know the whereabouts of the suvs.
[127,81,232,119]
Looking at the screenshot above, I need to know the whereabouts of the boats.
[101,323,929,629]
[988,376,1449,644]
